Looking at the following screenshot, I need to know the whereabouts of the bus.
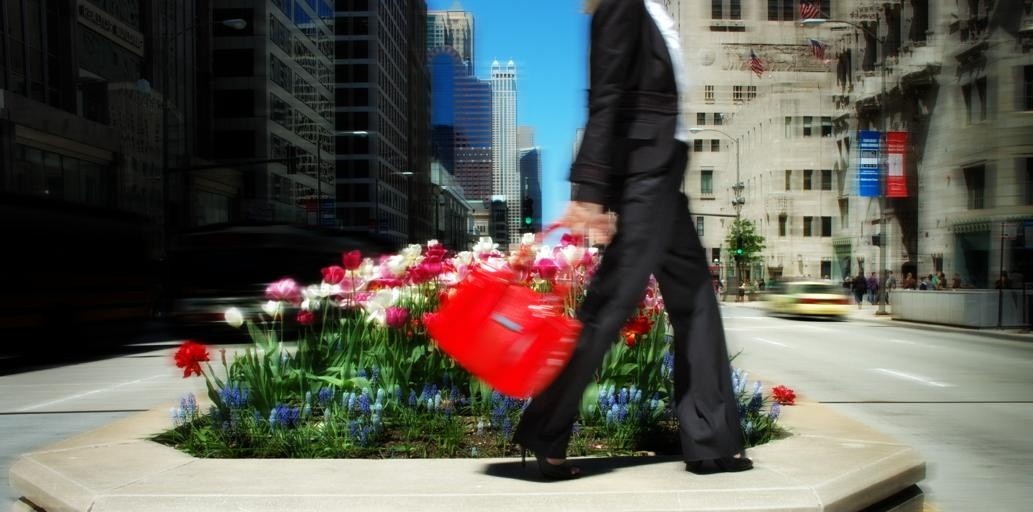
[175,222,379,337]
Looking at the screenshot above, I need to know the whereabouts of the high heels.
[684,441,755,476]
[508,433,590,481]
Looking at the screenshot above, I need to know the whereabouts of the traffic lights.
[524,198,533,225]
[736,238,742,255]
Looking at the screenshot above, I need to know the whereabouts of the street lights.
[800,15,890,320]
[686,125,744,288]
[713,258,724,285]
[374,171,414,256]
[315,129,368,226]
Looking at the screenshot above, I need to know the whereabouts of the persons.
[841,268,1010,309]
[711,275,765,302]
[511,0,754,472]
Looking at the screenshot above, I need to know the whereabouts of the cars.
[755,279,853,320]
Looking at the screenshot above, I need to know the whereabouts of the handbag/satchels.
[416,264,578,400]
